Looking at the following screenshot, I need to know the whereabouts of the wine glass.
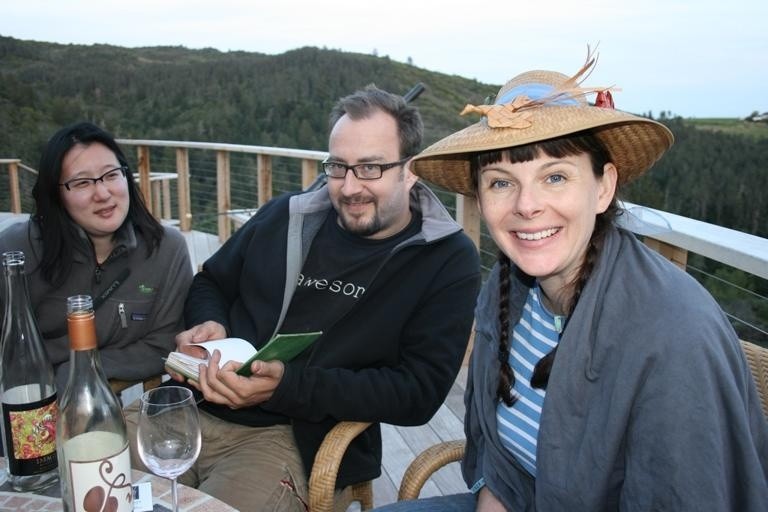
[133,386,204,512]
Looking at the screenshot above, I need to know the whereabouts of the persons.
[85,83,483,511]
[0,120,195,461]
[364,69,767,510]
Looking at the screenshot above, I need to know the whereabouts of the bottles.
[0,251,58,491]
[57,293,132,510]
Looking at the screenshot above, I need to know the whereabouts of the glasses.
[322,156,409,179]
[58,166,128,191]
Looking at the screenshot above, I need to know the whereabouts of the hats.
[407,70,675,198]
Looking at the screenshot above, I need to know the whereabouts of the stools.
[100,369,163,412]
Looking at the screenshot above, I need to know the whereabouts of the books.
[162,328,324,387]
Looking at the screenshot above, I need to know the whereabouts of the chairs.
[308,393,380,510]
[397,338,768,504]
